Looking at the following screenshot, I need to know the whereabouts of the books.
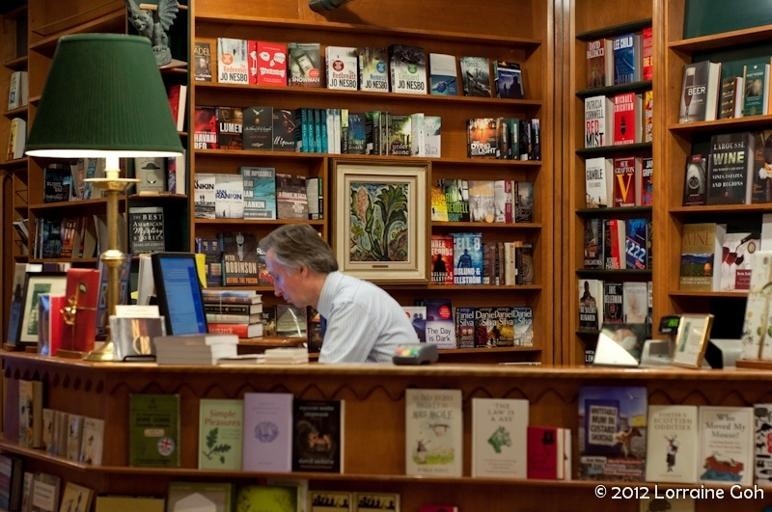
[5,118,26,160]
[195,37,425,219]
[133,157,165,193]
[170,81,188,131]
[167,149,186,195]
[8,72,29,109]
[1,159,110,511]
[154,330,241,366]
[109,382,772,511]
[427,27,771,362]
[196,230,306,341]
[129,205,166,257]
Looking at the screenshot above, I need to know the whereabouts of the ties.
[319,313,327,337]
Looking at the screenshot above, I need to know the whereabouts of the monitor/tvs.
[153,249,211,336]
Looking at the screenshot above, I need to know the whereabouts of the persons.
[400,305,427,343]
[411,312,419,335]
[419,313,426,342]
[259,222,420,363]
